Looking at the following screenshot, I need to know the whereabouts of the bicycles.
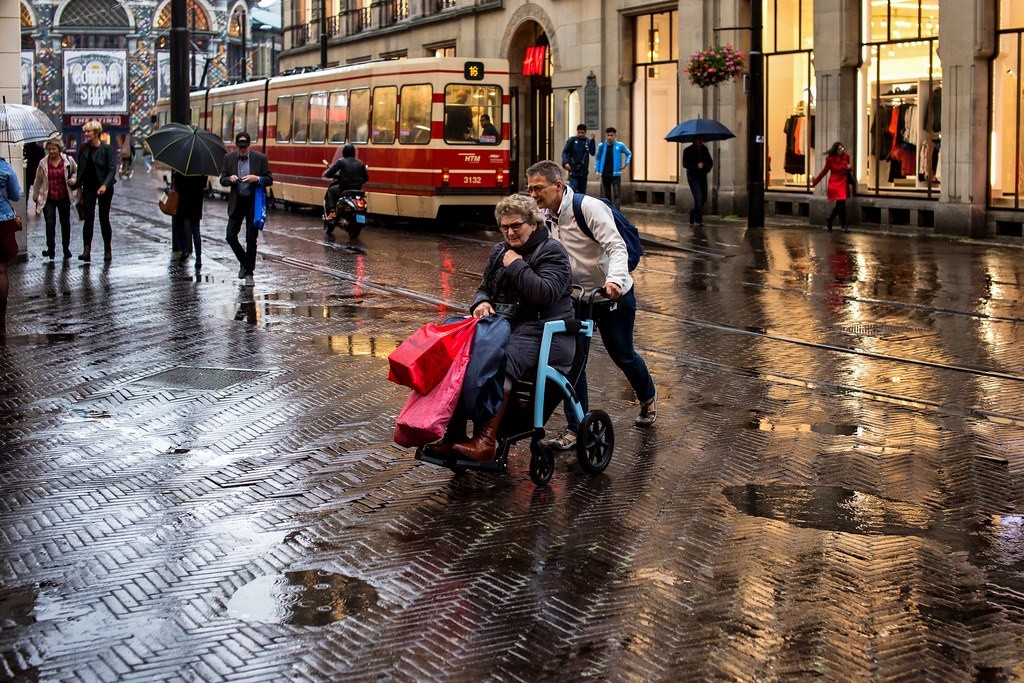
[117,149,136,180]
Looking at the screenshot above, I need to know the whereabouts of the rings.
[480,315,484,318]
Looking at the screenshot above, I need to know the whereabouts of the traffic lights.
[154,33,211,52]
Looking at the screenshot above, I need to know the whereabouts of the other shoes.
[179,252,189,262]
[238,264,245,279]
[327,212,336,219]
[104,249,112,261]
[245,276,256,286]
[146,167,152,173]
[62,247,73,257]
[41,248,56,259]
[842,225,850,233]
[78,251,91,262]
[688,209,704,226]
[824,219,833,232]
[194,257,203,267]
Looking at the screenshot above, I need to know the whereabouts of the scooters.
[322,159,369,239]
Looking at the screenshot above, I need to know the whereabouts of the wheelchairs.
[413,284,617,487]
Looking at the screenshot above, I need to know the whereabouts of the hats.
[235,132,251,146]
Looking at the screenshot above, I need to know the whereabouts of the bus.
[151,56,511,219]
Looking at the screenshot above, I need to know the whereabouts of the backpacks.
[572,192,642,272]
[121,144,131,158]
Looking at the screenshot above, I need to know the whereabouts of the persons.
[561,124,596,195]
[77,120,117,262]
[24,136,78,259]
[444,90,473,141]
[464,114,499,143]
[593,127,632,211]
[322,144,369,220]
[682,136,714,225]
[120,134,135,171]
[812,141,853,232]
[424,193,577,463]
[172,170,208,268]
[0,156,22,317]
[219,132,274,286]
[525,159,658,427]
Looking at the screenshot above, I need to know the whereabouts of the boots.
[453,392,512,463]
[424,411,467,457]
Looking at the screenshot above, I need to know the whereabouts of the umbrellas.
[663,114,736,143]
[0,95,61,165]
[144,122,228,177]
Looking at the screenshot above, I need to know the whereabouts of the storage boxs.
[388,328,453,396]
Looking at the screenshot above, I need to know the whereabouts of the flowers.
[684,42,747,89]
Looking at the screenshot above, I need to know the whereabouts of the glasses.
[522,179,558,192]
[497,220,531,232]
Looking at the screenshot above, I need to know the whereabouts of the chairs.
[296,126,430,143]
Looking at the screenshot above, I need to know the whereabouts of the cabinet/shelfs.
[871,77,942,188]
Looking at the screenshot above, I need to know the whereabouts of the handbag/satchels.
[76,181,86,221]
[254,177,267,232]
[159,191,179,216]
[846,154,856,186]
[393,315,486,449]
[14,215,24,233]
[563,161,588,177]
[385,316,480,394]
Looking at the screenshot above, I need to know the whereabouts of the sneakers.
[547,427,578,450]
[635,391,658,427]
